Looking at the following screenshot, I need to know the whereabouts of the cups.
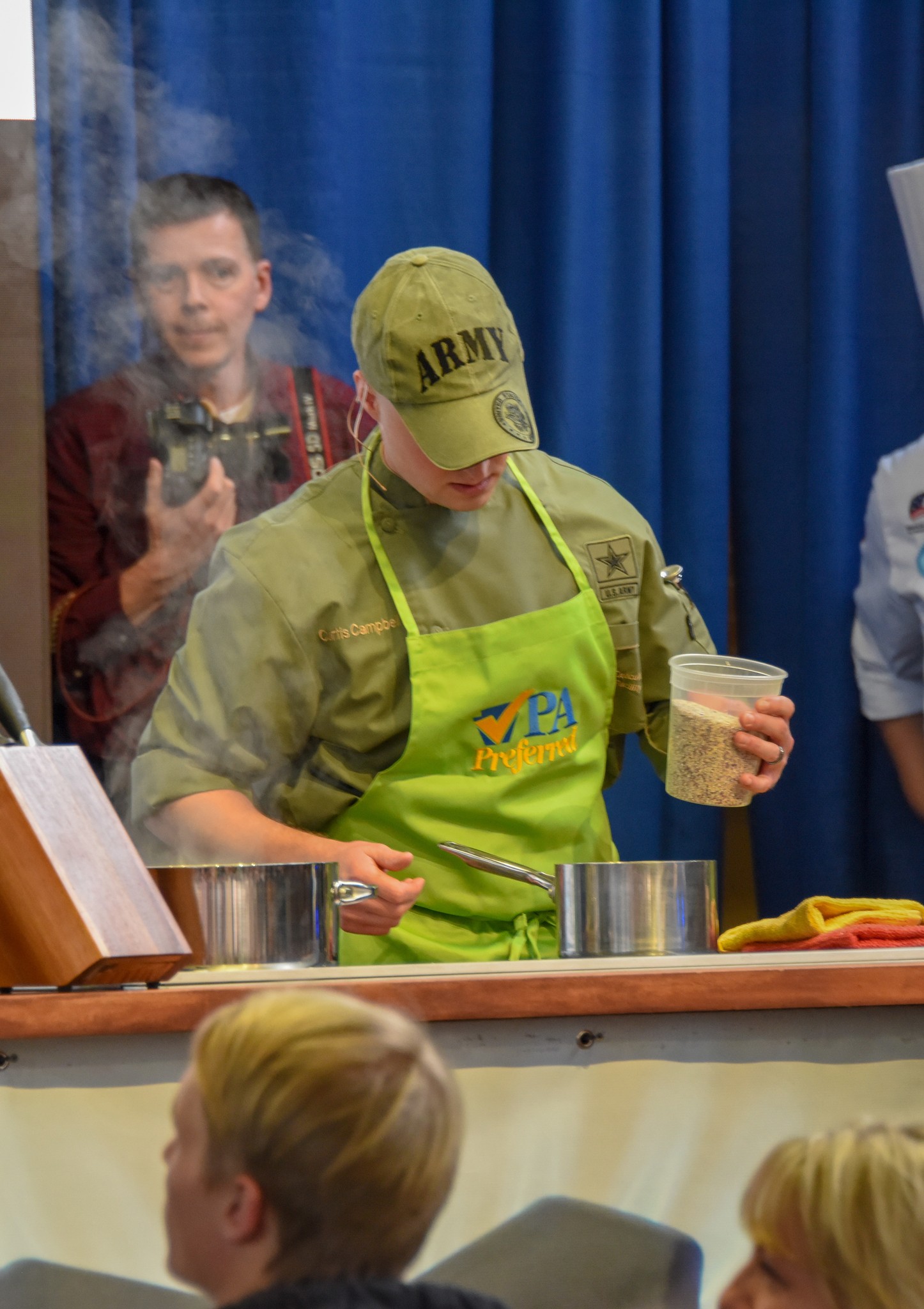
[660,648,787,809]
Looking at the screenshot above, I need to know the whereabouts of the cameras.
[147,397,292,516]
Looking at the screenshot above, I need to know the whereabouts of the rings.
[764,746,784,765]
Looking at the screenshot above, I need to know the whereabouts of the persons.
[845,152,923,838]
[716,1121,923,1309]
[44,171,384,819]
[123,243,804,965]
[159,989,509,1306]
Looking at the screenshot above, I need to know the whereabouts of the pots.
[145,856,379,973]
[434,840,718,960]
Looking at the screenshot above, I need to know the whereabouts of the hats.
[350,244,541,471]
